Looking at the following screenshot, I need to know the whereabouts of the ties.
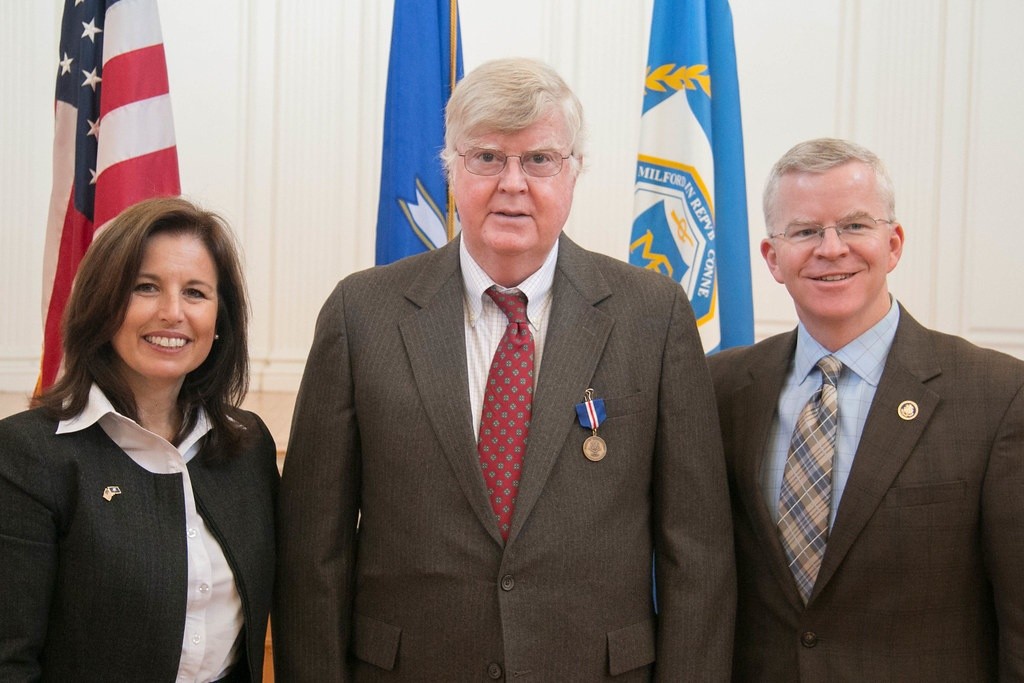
[478,288,535,538]
[777,355,843,604]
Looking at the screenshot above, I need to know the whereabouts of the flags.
[375,0,465,266]
[629,0,755,357]
[27,0,182,409]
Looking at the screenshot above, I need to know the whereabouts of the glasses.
[770,212,894,244]
[459,143,571,177]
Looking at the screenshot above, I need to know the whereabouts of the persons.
[270,60,738,683]
[705,137,1024,683]
[0,197,280,683]
